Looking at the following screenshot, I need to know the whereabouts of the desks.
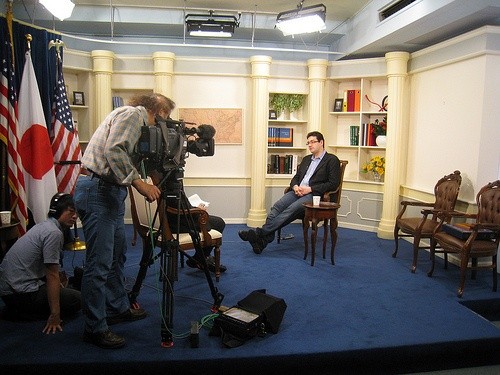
[0,218,20,263]
[302,201,341,266]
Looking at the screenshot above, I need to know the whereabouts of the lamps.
[274,0,326,36]
[184,10,241,37]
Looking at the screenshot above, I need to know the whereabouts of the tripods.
[130,168,224,347]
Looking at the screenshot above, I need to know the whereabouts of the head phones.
[48,194,70,217]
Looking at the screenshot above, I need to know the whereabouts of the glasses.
[306,140,322,145]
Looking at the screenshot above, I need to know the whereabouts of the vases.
[373,172,381,181]
[375,135,386,147]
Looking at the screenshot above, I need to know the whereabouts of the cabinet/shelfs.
[266,90,309,179]
[61,65,92,144]
[322,75,388,185]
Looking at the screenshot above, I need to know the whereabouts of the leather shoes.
[83,329,128,349]
[106,308,144,323]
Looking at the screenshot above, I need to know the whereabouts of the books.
[267,127,293,147]
[267,154,297,174]
[342,89,361,112]
[362,123,376,146]
[112,96,122,110]
[349,125,360,146]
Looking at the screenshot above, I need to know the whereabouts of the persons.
[71,93,177,344]
[0,193,83,334]
[166,178,228,272]
[238,131,341,254]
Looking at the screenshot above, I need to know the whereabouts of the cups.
[312,196,320,206]
[0,211,12,225]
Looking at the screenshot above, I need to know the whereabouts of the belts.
[79,168,102,179]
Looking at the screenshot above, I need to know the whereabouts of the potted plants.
[269,94,304,120]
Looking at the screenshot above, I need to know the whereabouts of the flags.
[0,33,84,236]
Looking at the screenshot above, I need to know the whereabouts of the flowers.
[360,156,385,176]
[371,116,387,136]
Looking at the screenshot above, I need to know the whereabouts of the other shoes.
[186,256,205,270]
[238,230,258,241]
[248,229,266,254]
[206,256,227,273]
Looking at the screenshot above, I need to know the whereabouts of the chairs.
[277,160,349,244]
[127,170,222,295]
[427,180,500,298]
[392,171,462,272]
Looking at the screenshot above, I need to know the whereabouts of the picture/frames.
[73,91,85,106]
[334,98,343,112]
[269,110,277,119]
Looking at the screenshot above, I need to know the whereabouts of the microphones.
[187,124,216,140]
